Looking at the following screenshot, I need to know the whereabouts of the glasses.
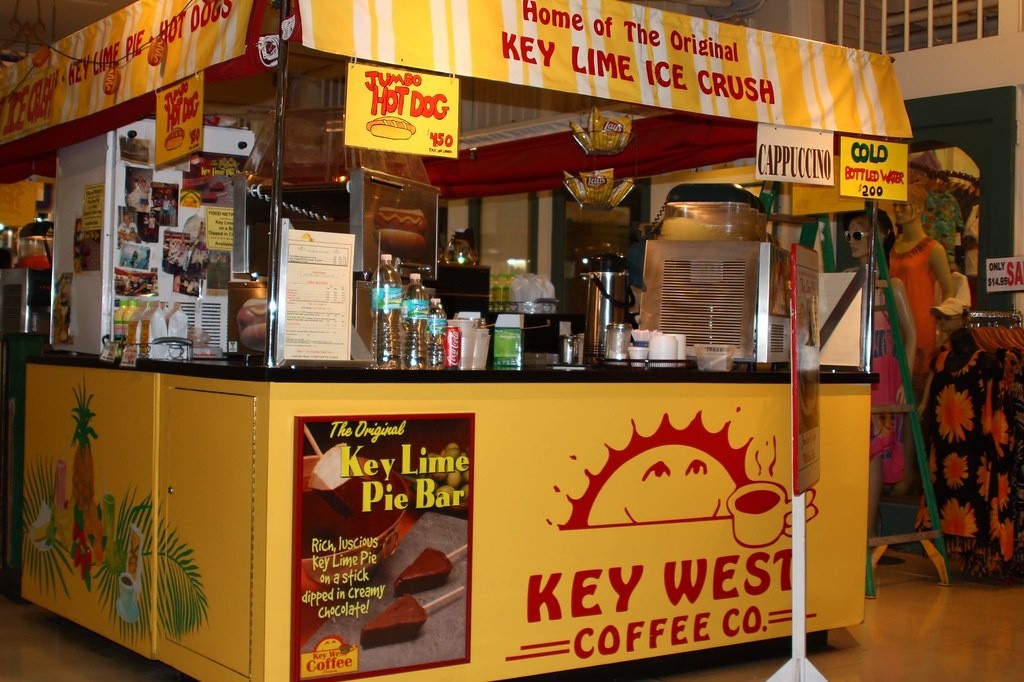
[935,314,962,321]
[843,230,869,241]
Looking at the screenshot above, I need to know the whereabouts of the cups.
[447,319,492,370]
[648,335,678,368]
[665,334,687,368]
[628,347,650,368]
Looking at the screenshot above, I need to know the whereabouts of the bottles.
[427,297,447,369]
[113,299,154,360]
[401,273,431,369]
[370,253,403,368]
[489,272,558,314]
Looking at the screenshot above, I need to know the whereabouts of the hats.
[928,297,970,316]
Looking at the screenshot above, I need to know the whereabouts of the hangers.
[966,324,1024,365]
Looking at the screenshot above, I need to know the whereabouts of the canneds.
[443,326,462,368]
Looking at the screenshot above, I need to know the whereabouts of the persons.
[0,249,13,268]
[917,296,971,422]
[838,209,915,550]
[883,181,955,494]
[53,167,203,347]
